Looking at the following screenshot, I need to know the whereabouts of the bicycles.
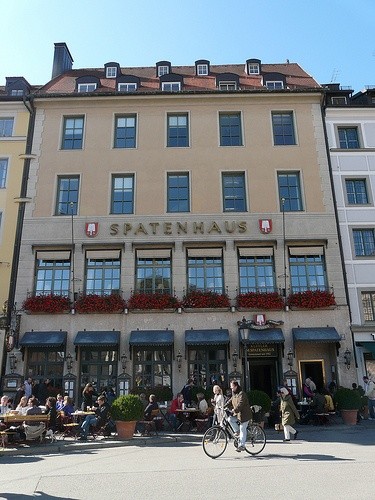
[202,408,267,459]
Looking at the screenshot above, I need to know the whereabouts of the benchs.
[0,414,50,448]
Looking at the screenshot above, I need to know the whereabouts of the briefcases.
[274,423,284,430]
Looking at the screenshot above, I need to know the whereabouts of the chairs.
[138,408,159,437]
[59,415,79,441]
[194,407,213,434]
[91,413,112,442]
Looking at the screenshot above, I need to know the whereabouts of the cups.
[87,406,90,411]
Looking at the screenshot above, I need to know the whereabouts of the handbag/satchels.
[206,407,213,414]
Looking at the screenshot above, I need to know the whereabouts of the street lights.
[239,316,249,393]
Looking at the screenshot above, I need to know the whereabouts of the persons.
[0,376,375,441]
[218,380,251,452]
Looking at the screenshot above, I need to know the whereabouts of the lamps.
[344,347,352,368]
[287,347,294,367]
[65,351,74,373]
[9,353,18,374]
[121,354,128,371]
[233,348,239,367]
[176,352,183,373]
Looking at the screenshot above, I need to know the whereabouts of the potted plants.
[360,396,369,420]
[334,387,364,426]
[111,393,147,440]
[246,389,272,430]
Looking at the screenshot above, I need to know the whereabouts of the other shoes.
[236,446,244,451]
[284,439,290,441]
[191,428,197,432]
[78,434,87,440]
[208,435,215,441]
[361,415,364,419]
[19,443,30,448]
[294,430,299,439]
[367,417,375,420]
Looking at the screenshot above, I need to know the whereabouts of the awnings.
[19,328,341,348]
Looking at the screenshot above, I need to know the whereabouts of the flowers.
[285,288,337,309]
[181,285,233,309]
[234,291,286,309]
[19,292,74,314]
[127,288,181,310]
[73,289,127,313]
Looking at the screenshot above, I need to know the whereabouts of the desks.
[160,406,173,428]
[176,407,198,434]
[298,402,313,412]
[71,411,96,442]
